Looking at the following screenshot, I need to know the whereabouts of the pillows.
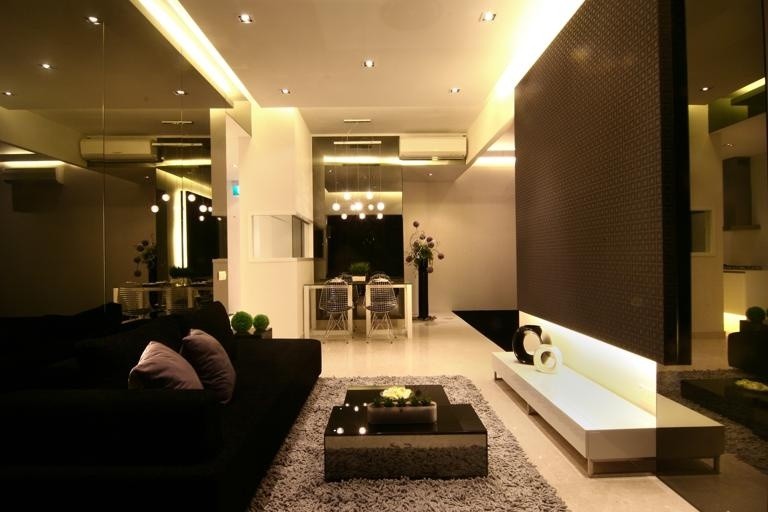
[128,328,236,404]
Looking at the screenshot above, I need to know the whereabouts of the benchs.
[657,391,726,474]
[493,310,657,478]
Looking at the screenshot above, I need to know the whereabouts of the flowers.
[406,221,444,272]
[134,236,163,277]
[148,269,160,306]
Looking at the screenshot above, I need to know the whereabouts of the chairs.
[319,272,397,344]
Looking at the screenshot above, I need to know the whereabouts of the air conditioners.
[80,134,157,162]
[399,135,467,160]
[1,160,63,183]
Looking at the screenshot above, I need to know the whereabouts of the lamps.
[150,120,212,222]
[333,119,386,222]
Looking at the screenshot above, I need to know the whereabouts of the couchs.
[0,302,321,512]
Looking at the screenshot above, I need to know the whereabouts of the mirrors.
[1,0,103,319]
[104,0,227,328]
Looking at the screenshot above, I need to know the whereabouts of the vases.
[418,258,428,320]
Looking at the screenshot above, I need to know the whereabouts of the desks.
[113,281,213,317]
[365,284,412,338]
[304,285,353,340]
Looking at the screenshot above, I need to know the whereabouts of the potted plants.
[169,266,199,285]
[366,395,438,424]
[231,310,272,340]
[740,306,767,333]
[349,262,371,283]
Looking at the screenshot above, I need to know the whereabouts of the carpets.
[659,368,768,472]
[249,375,571,512]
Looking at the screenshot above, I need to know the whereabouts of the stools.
[728,332,767,372]
